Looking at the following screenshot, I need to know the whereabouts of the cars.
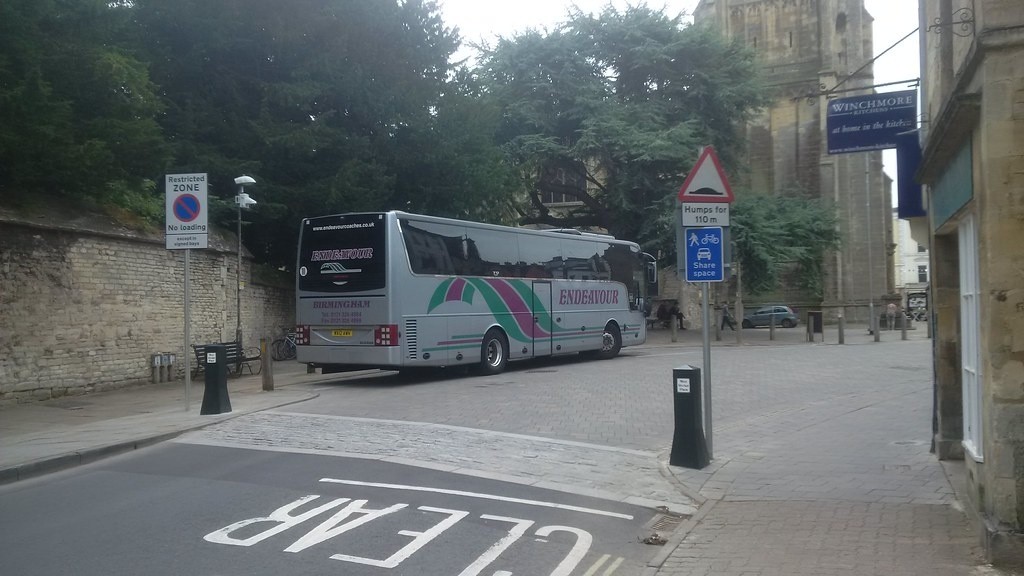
[742,304,800,329]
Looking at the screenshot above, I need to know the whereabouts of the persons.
[909,298,916,307]
[670,301,687,329]
[646,296,652,316]
[886,302,897,330]
[917,304,926,319]
[658,299,672,329]
[721,301,736,331]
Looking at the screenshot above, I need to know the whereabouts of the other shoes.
[680,327,686,330]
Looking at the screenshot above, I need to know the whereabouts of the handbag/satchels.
[730,317,738,325]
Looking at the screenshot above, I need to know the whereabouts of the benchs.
[191,341,263,379]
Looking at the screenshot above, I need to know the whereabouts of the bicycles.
[272,326,295,360]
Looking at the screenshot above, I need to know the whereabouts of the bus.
[295,210,657,376]
[907,290,927,316]
